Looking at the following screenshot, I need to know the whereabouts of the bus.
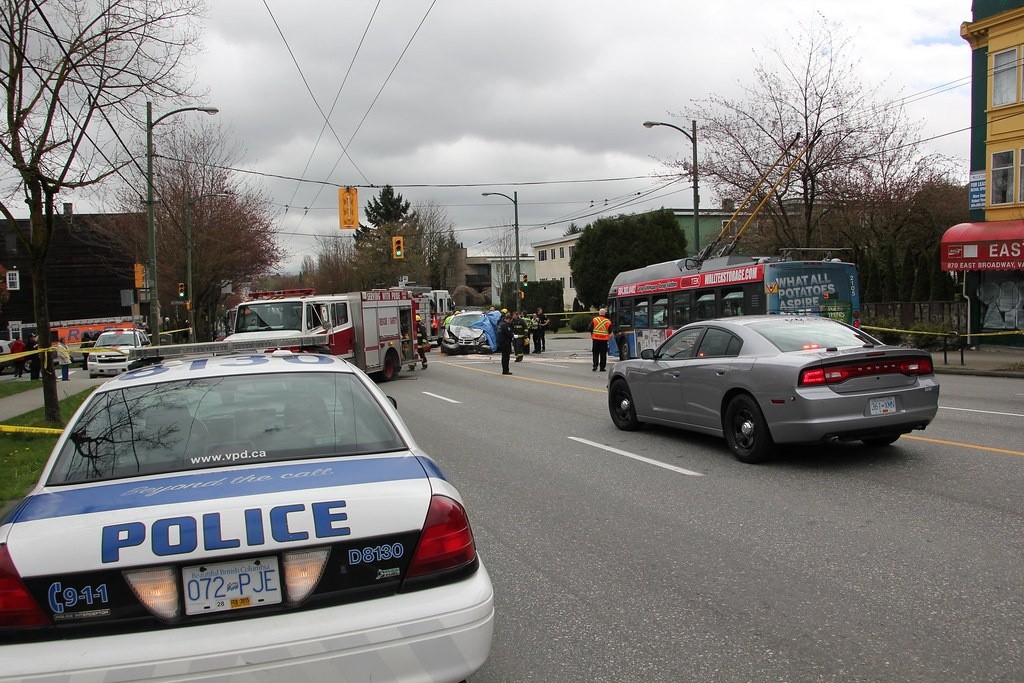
[605,129,861,361]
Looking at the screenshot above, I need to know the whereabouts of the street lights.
[146,102,218,346]
[482,191,520,315]
[187,190,228,342]
[643,120,700,256]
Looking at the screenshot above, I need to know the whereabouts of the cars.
[440,310,494,354]
[606,314,940,465]
[1,332,495,682]
[87,329,152,379]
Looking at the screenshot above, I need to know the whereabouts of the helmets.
[416,315,421,321]
[501,309,508,314]
[454,310,460,315]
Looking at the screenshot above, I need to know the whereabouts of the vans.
[0,321,147,373]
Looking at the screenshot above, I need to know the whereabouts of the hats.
[598,309,607,315]
[28,333,37,339]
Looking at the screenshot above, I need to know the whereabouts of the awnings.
[941,219,1024,272]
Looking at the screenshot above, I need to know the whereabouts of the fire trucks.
[212,275,456,386]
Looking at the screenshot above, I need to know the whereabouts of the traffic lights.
[393,237,404,260]
[523,274,528,287]
[178,283,185,298]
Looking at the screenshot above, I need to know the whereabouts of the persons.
[80,328,102,370]
[10,333,42,381]
[587,308,613,372]
[441,311,460,353]
[408,315,428,371]
[490,307,549,375]
[57,338,72,381]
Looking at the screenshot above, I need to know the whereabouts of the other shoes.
[600,368,606,371]
[515,357,522,362]
[523,348,545,355]
[502,371,512,375]
[421,365,428,370]
[592,366,597,371]
